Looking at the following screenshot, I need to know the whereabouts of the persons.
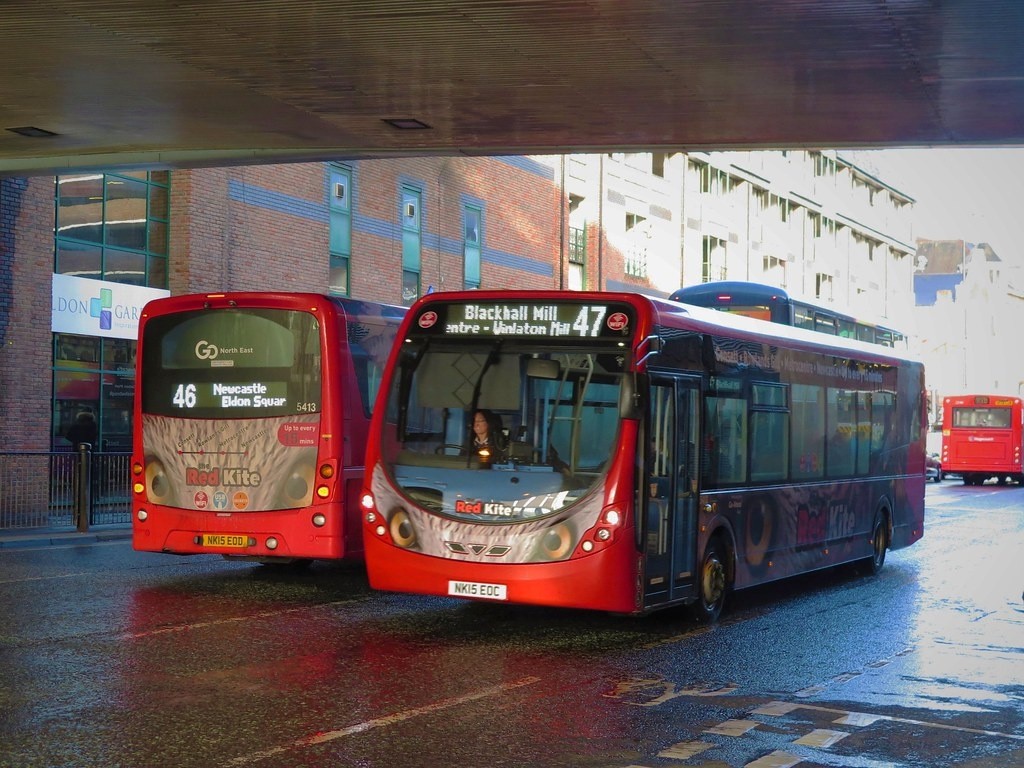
[65,407,98,452]
[459,408,504,458]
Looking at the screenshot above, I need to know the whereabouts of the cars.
[925,455,942,482]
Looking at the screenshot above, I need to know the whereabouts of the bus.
[668,282,909,353]
[940,394,1024,487]
[129,289,441,564]
[358,294,928,623]
[53,333,117,409]
